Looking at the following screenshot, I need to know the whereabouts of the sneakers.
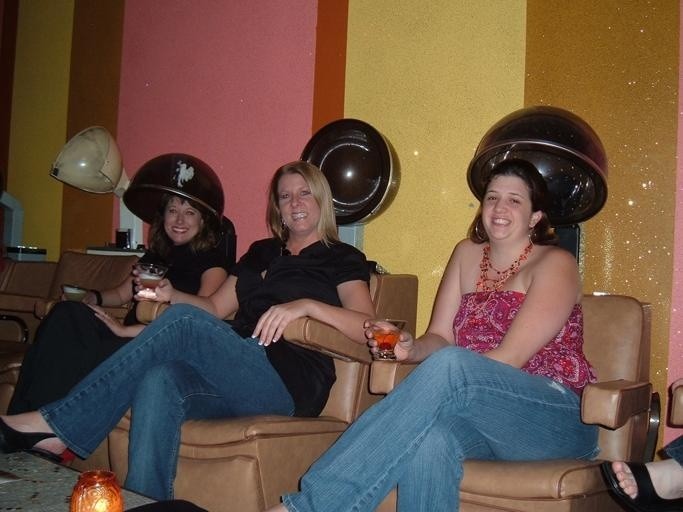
[0,415,65,464]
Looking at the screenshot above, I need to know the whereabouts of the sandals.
[599,460,683,512]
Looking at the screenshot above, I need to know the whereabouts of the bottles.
[70,469,124,512]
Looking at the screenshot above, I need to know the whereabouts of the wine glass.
[366,319,407,361]
[134,262,169,300]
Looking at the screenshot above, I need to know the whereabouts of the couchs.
[0,248,238,481]
[109,270,419,509]
[370,291,662,509]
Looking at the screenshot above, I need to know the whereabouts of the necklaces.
[473,238,538,294]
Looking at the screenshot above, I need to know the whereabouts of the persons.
[602,430,683,511]
[0,161,377,501]
[0,190,231,468]
[263,155,604,512]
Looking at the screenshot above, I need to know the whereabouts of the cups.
[62,283,88,302]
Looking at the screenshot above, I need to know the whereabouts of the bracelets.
[89,289,103,307]
